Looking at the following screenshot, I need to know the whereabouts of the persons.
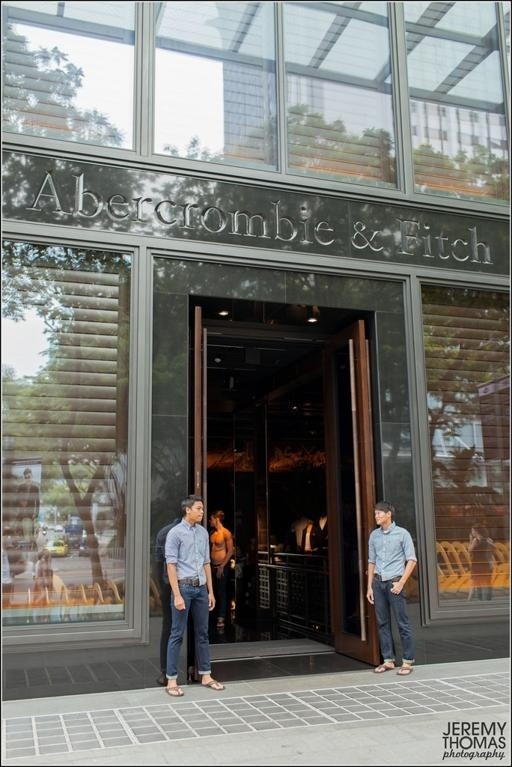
[365,502,420,676]
[154,498,197,686]
[468,525,495,600]
[208,509,233,629]
[163,495,226,697]
[301,513,317,552]
[17,466,40,524]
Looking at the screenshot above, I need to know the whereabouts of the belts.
[374,573,402,582]
[178,579,200,587]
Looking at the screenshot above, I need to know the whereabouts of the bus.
[65,516,83,549]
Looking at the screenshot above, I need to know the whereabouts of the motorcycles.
[43,525,49,536]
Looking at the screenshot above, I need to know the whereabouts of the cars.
[54,525,63,533]
[42,540,69,557]
[79,546,91,557]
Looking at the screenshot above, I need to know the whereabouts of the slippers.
[165,685,184,696]
[201,677,225,690]
[374,660,394,673]
[397,663,414,676]
